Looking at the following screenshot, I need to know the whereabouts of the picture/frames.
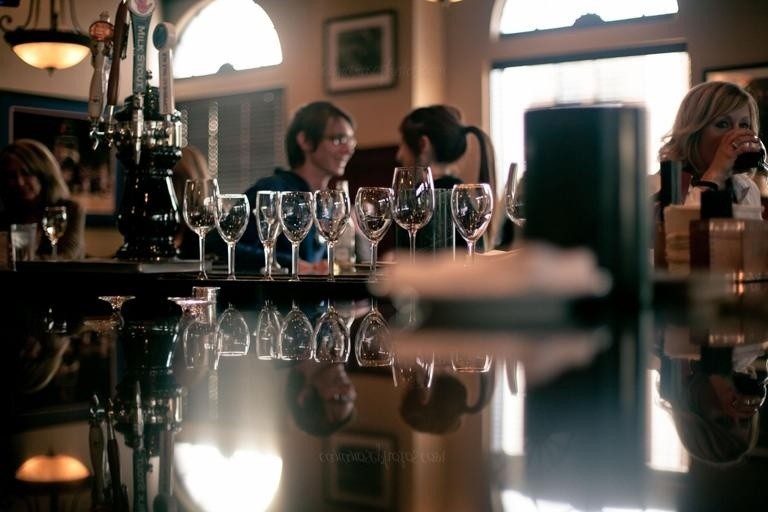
[9,105,118,216]
[323,8,399,97]
[323,427,399,512]
[701,63,768,155]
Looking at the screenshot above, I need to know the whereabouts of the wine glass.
[40,204,70,264]
[390,168,436,267]
[501,162,529,228]
[451,182,495,255]
[277,191,316,282]
[315,189,351,283]
[355,187,394,282]
[210,191,250,283]
[182,177,223,281]
[96,292,493,389]
[258,190,285,283]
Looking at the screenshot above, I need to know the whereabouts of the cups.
[9,222,37,264]
[726,376,766,416]
[727,134,765,171]
[42,306,69,337]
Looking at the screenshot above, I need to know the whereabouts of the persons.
[169,144,315,271]
[4,335,72,394]
[224,101,357,263]
[654,346,768,471]
[286,363,358,439]
[395,103,496,249]
[399,364,495,436]
[653,80,767,266]
[1,139,87,261]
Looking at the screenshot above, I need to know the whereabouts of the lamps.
[0,0,91,76]
[1,436,93,512]
